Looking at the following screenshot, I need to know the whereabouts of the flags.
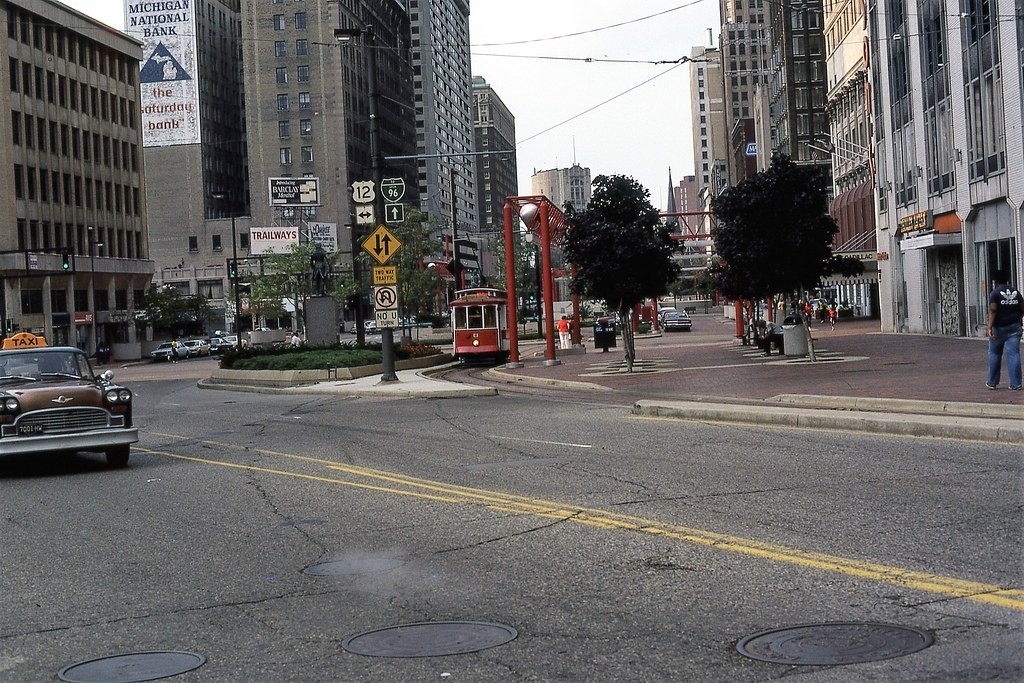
[810,137,834,165]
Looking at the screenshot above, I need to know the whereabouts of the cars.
[350,310,450,335]
[0,331,140,478]
[209,334,248,356]
[150,341,191,362]
[810,298,829,316]
[255,327,303,344]
[595,306,692,332]
[182,340,211,358]
[740,300,802,319]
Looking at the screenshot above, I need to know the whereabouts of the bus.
[449,287,512,365]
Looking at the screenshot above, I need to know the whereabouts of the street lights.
[332,24,398,380]
[209,189,242,348]
[91,241,104,366]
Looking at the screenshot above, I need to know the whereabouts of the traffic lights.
[230,263,235,275]
[6,319,13,333]
[529,292,534,301]
[63,253,69,269]
[12,323,19,333]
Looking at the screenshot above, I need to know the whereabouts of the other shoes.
[986,381,996,390]
[1008,385,1022,390]
[761,352,771,357]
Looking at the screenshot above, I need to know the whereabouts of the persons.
[818,299,825,323]
[168,337,178,363]
[291,333,301,348]
[829,308,836,331]
[310,243,332,295]
[829,299,838,309]
[803,301,813,330]
[985,270,1024,390]
[557,316,570,349]
[779,300,785,310]
[758,320,783,357]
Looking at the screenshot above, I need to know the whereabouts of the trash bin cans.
[781,314,809,355]
[593,320,617,348]
